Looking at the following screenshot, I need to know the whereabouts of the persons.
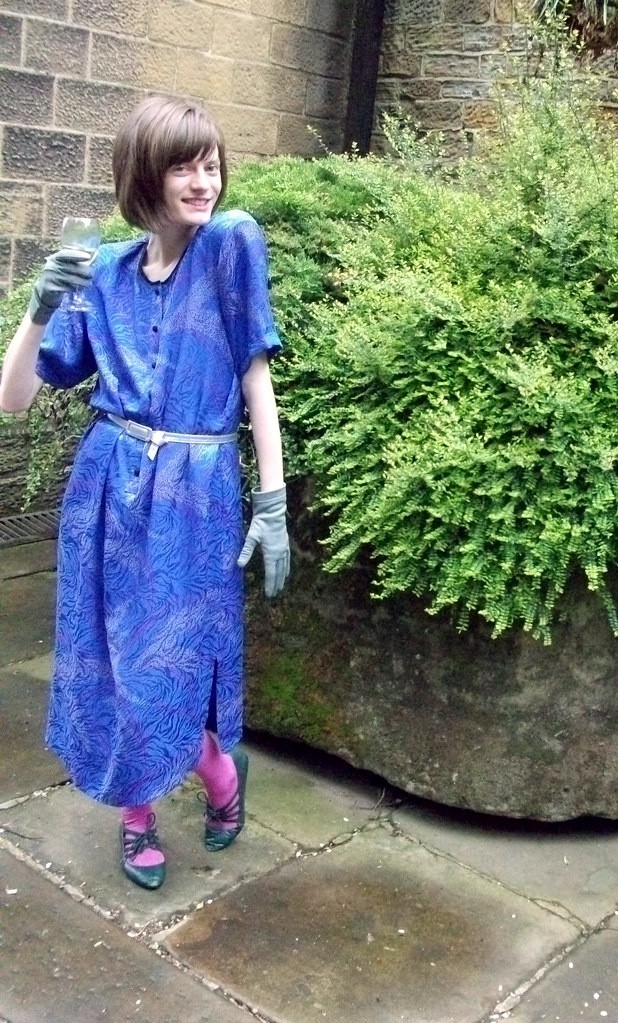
[0,94,291,891]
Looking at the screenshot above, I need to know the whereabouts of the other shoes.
[120,812,165,889]
[197,751,249,851]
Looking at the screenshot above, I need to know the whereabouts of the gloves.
[237,483,290,598]
[29,250,92,325]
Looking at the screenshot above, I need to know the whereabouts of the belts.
[106,412,239,460]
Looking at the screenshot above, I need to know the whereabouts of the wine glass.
[59,216,101,313]
